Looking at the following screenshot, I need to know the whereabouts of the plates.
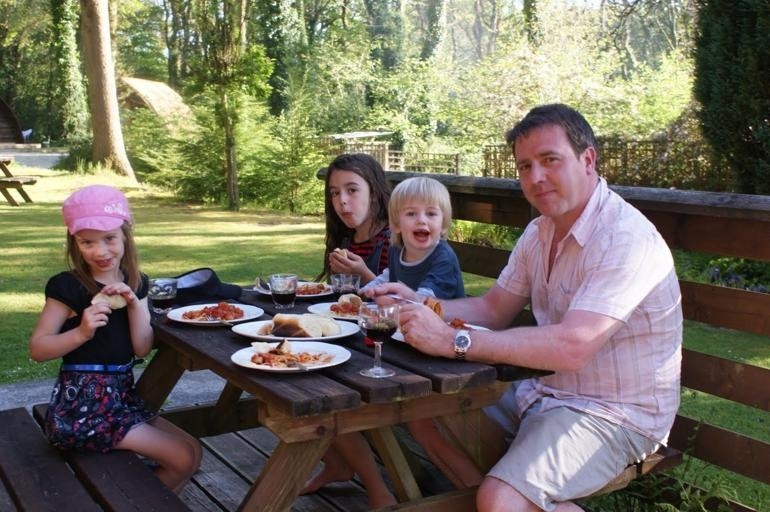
[307,302,379,319]
[231,320,360,340]
[167,304,264,325]
[231,341,352,373]
[253,282,334,296]
[391,321,492,343]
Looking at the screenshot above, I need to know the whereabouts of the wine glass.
[358,302,399,379]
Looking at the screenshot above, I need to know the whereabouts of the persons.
[30,185,203,497]
[297,176,465,512]
[374,103,683,512]
[300,153,391,497]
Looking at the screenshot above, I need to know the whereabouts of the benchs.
[572,444,682,502]
[0,402,194,512]
[0,159,37,206]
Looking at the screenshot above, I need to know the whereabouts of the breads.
[257,312,341,338]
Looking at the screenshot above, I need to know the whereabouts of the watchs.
[454,328,472,362]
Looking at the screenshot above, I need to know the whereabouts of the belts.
[61,358,145,373]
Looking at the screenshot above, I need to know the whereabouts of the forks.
[206,315,234,326]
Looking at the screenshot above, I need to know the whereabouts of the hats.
[177,269,241,307]
[62,185,132,235]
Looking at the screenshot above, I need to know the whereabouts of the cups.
[269,274,297,309]
[331,274,361,298]
[151,278,178,314]
